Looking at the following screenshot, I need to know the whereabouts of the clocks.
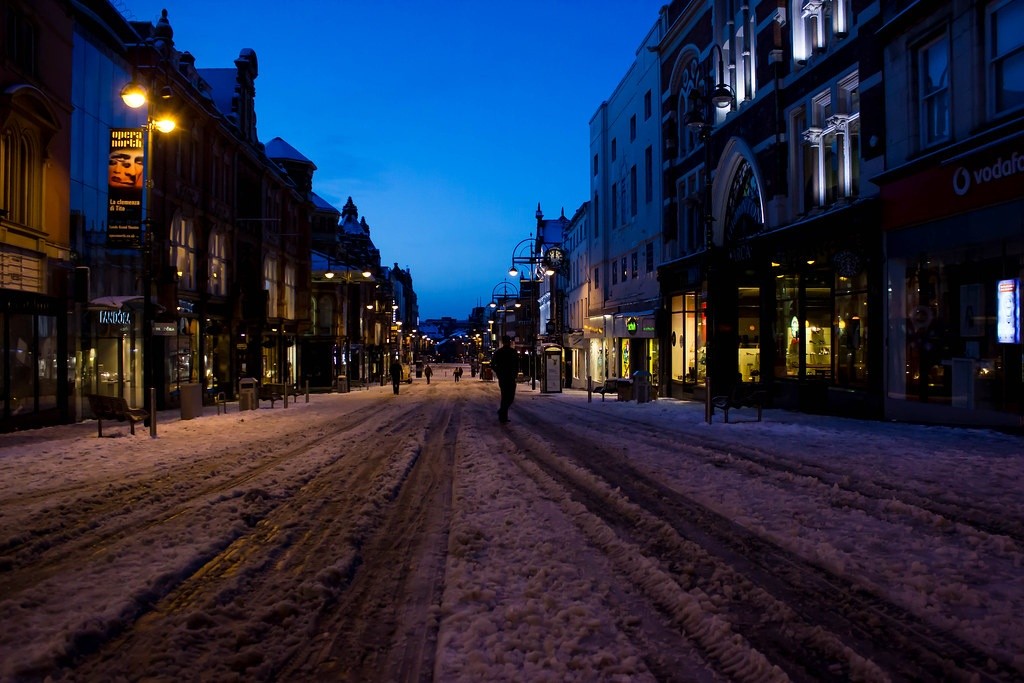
[543,246,565,267]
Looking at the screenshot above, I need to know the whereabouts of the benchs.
[592,379,617,402]
[87,393,148,437]
[348,378,370,391]
[262,381,296,408]
[712,372,772,424]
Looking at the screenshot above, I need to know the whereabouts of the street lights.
[367,283,431,387]
[506,232,556,392]
[488,277,523,347]
[120,62,180,427]
[324,270,372,393]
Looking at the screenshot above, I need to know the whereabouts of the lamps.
[796,30,847,66]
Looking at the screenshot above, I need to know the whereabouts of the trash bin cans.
[632,369,652,405]
[237,376,260,413]
[615,377,632,402]
[337,374,349,395]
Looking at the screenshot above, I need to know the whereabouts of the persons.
[453,367,463,383]
[425,364,433,384]
[389,359,403,394]
[491,335,519,423]
